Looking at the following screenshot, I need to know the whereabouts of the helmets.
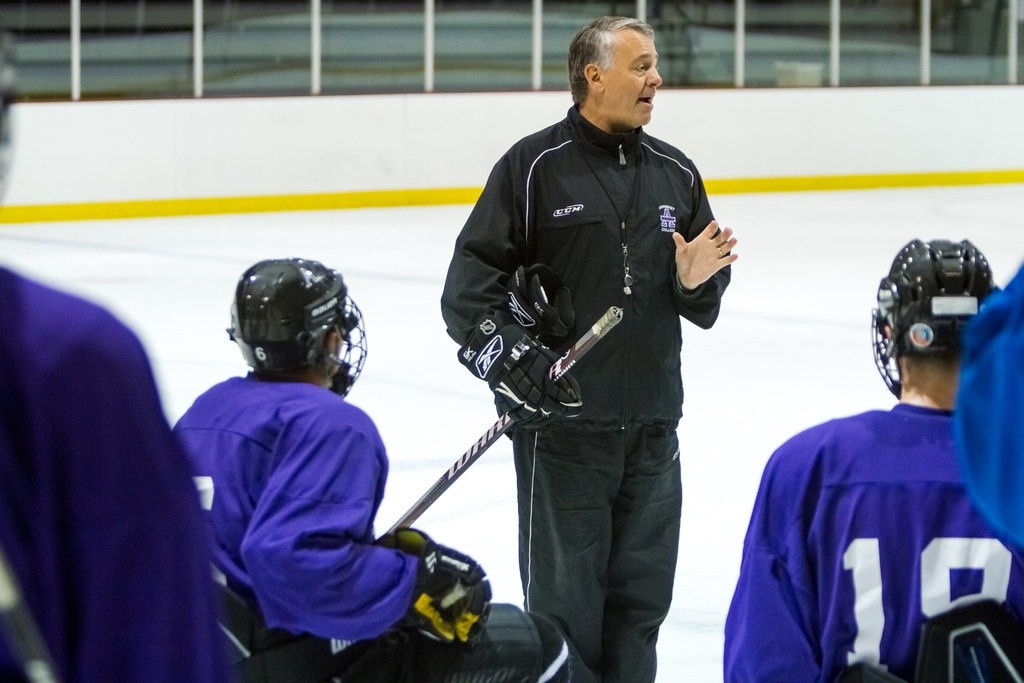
[227,256,367,397]
[873,238,993,395]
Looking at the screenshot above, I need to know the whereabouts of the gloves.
[406,544,496,647]
[505,258,574,342]
[457,310,588,431]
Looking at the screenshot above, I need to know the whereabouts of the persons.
[0,37,240,683]
[723,237,1024,683]
[439,14,738,683]
[170,259,572,683]
